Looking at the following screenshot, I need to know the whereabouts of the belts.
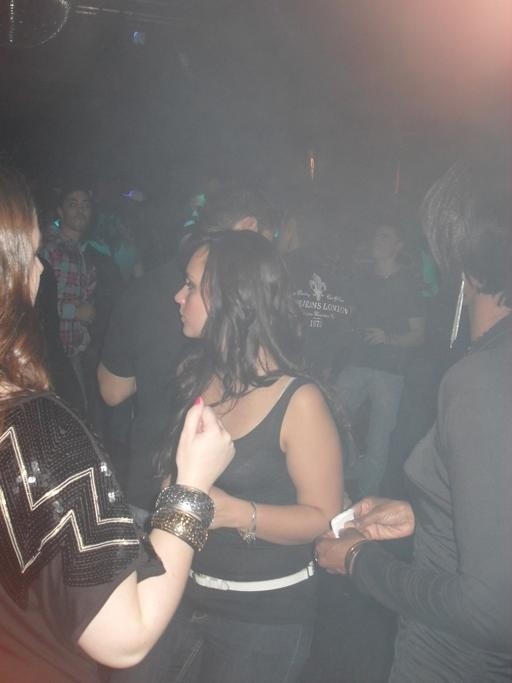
[189,561,315,592]
